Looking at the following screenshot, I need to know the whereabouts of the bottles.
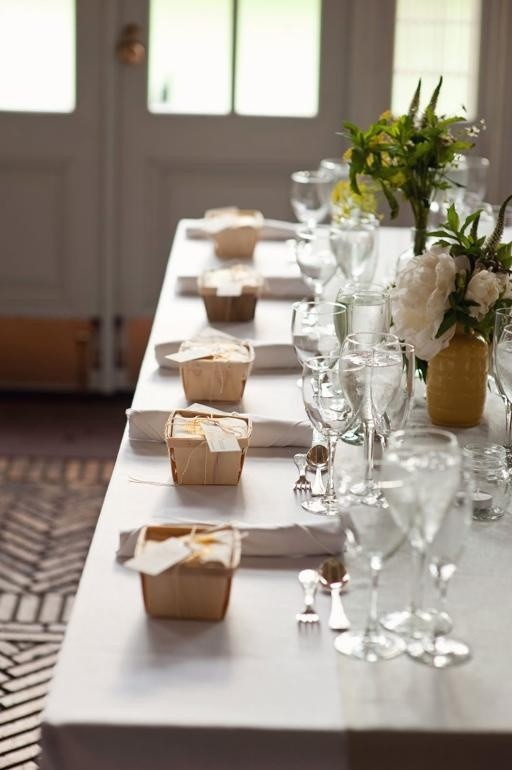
[453,440,510,522]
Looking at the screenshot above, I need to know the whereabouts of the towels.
[116,521,347,563]
[181,219,297,240]
[124,408,314,452]
[176,273,312,299]
[151,342,304,369]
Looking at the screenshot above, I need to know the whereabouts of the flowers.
[387,203,512,358]
[334,79,488,222]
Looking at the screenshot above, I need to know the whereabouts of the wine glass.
[288,156,473,676]
[492,309,512,451]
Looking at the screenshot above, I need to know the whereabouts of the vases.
[424,317,490,429]
